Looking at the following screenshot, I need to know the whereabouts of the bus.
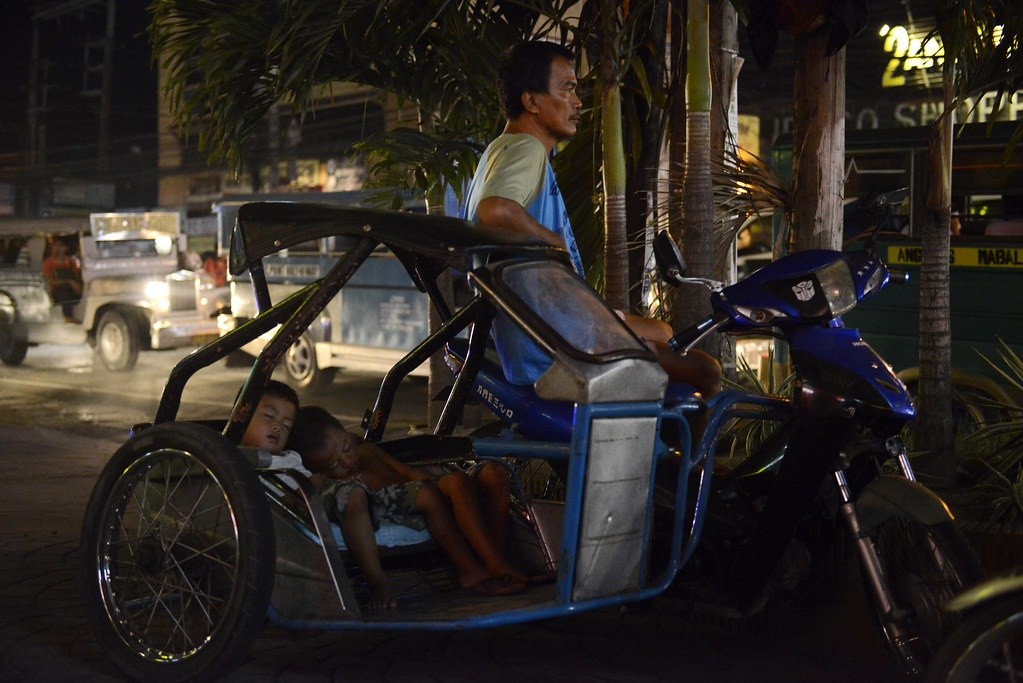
[746,78,1023,467]
[213,185,447,397]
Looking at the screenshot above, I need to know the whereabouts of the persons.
[842,174,895,241]
[230,380,532,611]
[454,40,731,477]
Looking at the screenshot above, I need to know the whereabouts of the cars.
[0,212,236,376]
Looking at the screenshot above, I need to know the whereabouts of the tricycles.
[76,201,984,683]
